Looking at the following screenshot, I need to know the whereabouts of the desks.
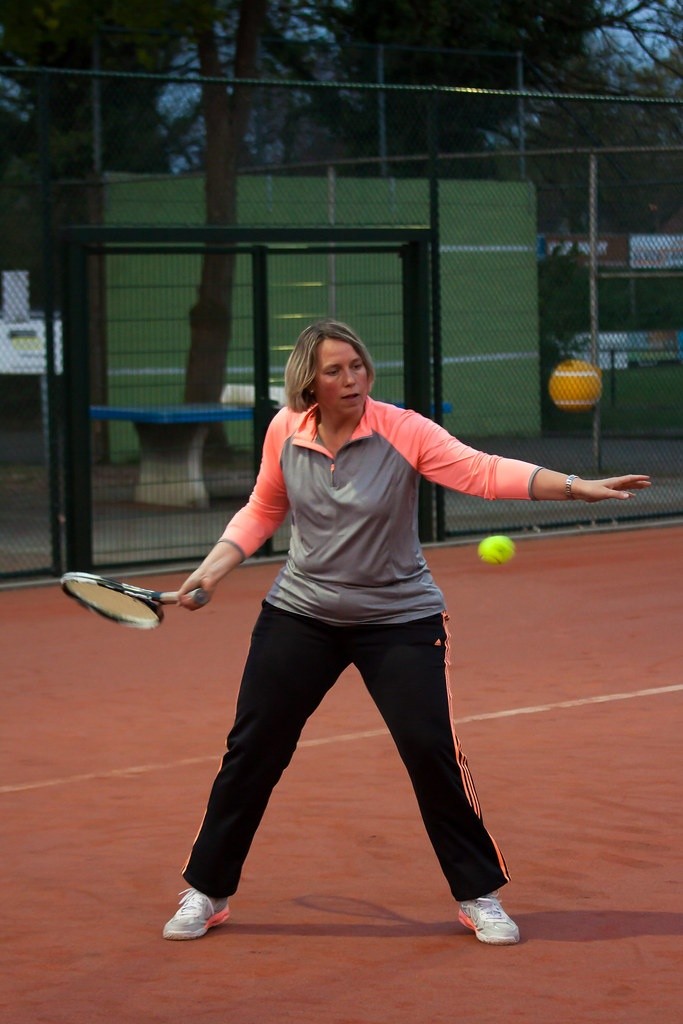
[90,401,452,508]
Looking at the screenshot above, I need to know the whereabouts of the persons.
[160,319,652,948]
[547,235,586,258]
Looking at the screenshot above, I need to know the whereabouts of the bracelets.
[564,475,581,501]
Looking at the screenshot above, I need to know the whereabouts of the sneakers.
[163,887,230,941]
[458,892,519,945]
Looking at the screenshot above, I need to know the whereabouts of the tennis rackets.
[59,570,208,632]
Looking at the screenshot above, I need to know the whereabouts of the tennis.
[475,533,516,565]
